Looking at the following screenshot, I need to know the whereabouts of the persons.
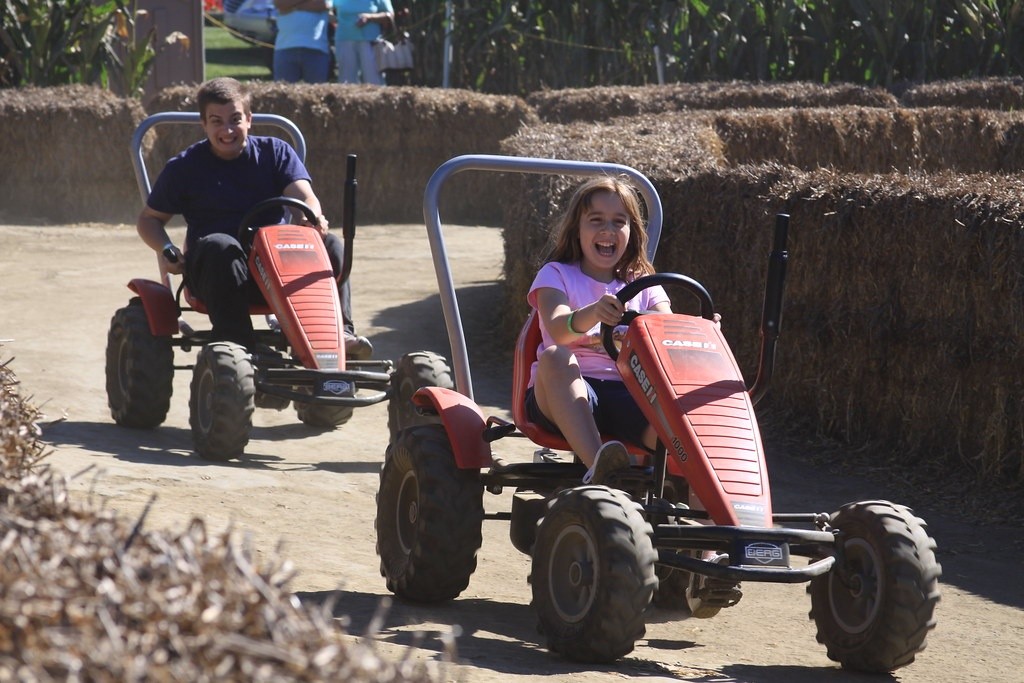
[136,77,373,363]
[334,0,394,85]
[395,32,415,85]
[272,0,332,82]
[524,173,739,617]
[374,37,398,83]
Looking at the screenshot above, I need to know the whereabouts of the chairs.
[512,304,654,459]
[183,239,273,316]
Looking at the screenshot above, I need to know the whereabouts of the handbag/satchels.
[371,12,414,72]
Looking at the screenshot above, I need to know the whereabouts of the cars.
[221,0,277,50]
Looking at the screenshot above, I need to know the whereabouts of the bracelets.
[567,311,586,336]
[162,243,174,250]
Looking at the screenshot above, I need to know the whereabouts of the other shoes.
[583,440,630,486]
[685,554,732,620]
[344,332,372,359]
